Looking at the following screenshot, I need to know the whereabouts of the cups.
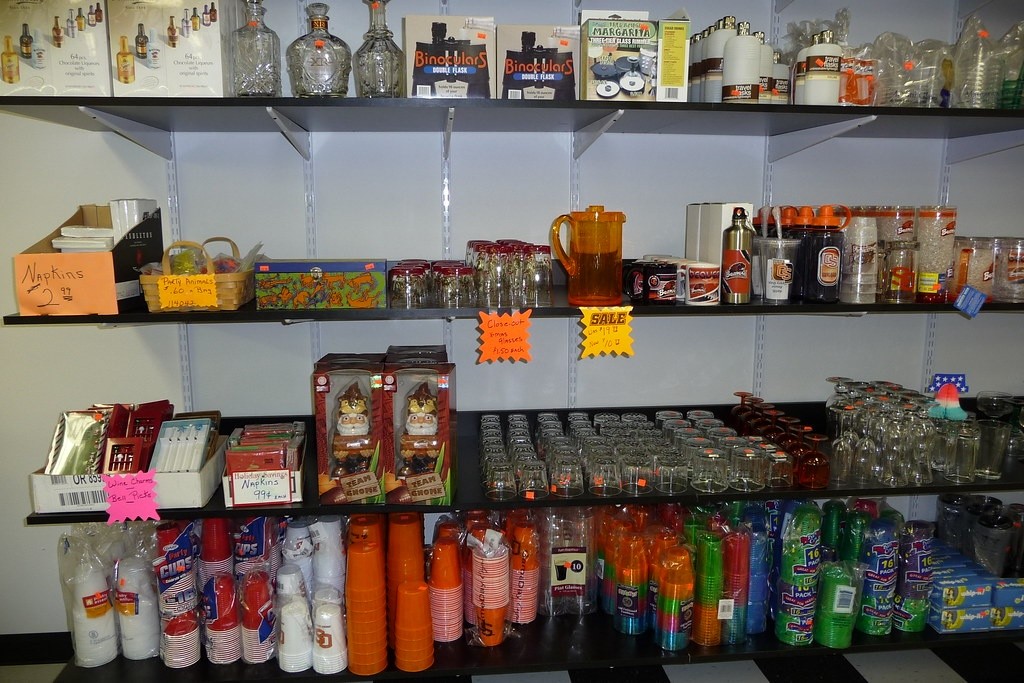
[933,418,1011,481]
[76,490,1024,676]
[388,240,552,308]
[832,206,1024,305]
[752,237,801,304]
[976,393,1015,416]
[479,410,794,499]
[623,255,719,306]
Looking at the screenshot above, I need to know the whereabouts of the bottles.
[353,0,405,99]
[285,3,352,97]
[721,207,754,304]
[231,0,280,96]
[688,15,841,106]
[0,1,217,83]
[753,205,853,301]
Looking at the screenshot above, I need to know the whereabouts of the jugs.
[552,206,627,306]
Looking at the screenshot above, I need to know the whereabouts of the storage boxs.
[685,201,754,266]
[311,344,458,508]
[399,8,691,105]
[922,543,1024,635]
[28,434,230,508]
[13,204,164,315]
[221,435,309,508]
[253,259,389,310]
[0,0,249,100]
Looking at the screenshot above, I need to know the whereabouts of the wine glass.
[1019,407,1024,459]
[732,376,937,487]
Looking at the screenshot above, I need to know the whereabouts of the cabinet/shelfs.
[0,0,1024,683]
